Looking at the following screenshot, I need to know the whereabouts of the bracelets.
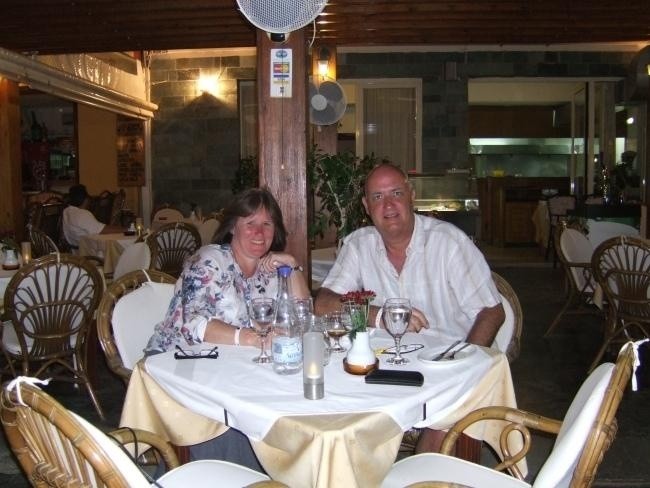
[374,306,385,331]
[233,324,243,346]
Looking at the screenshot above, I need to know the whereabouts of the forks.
[444,341,473,360]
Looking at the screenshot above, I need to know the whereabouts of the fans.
[308,73,347,126]
[236,0,331,42]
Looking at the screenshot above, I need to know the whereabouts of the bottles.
[269,265,303,374]
[2,264,19,270]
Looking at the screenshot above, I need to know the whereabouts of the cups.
[340,301,370,333]
[20,241,32,267]
[136,224,143,240]
[292,292,331,401]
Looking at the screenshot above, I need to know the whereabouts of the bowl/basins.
[123,231,135,235]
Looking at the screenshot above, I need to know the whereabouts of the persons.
[141,184,317,487]
[59,184,132,258]
[310,155,508,458]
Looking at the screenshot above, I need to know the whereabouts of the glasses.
[174,344,219,359]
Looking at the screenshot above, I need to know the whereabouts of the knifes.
[433,340,461,361]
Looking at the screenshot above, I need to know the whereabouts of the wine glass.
[248,298,277,363]
[384,298,413,366]
[326,311,349,355]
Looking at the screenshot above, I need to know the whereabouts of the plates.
[418,344,478,365]
